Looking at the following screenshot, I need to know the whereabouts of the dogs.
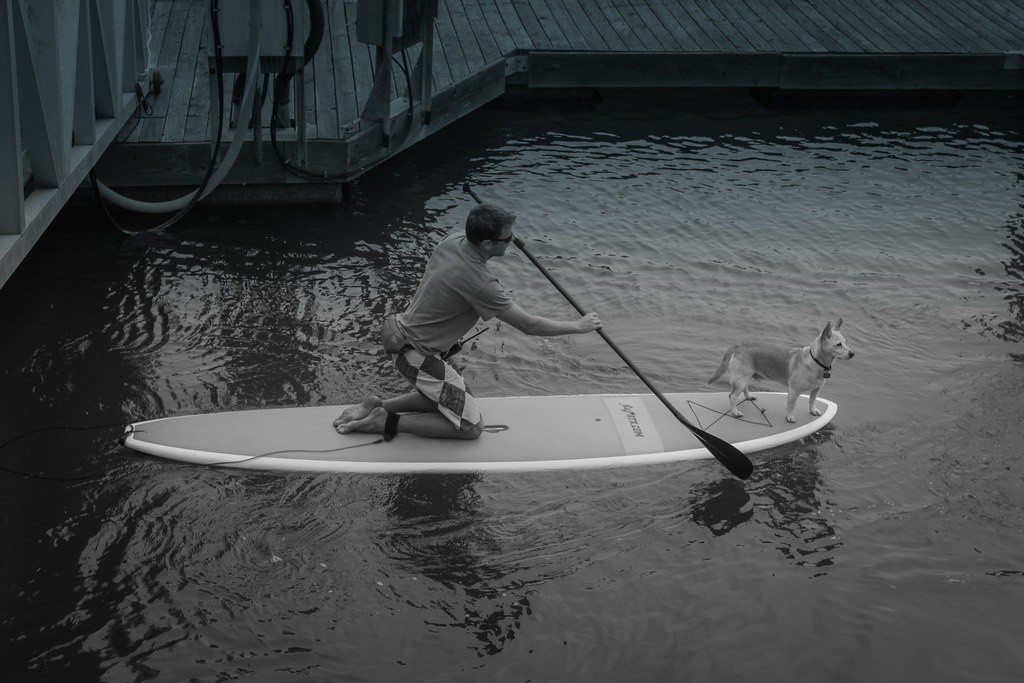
[706,317,855,423]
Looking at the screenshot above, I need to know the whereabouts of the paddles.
[463,182,753,479]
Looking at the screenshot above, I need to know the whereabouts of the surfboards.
[124,391,838,474]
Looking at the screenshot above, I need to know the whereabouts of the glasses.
[487,232,514,244]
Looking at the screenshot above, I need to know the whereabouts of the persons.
[331,205,604,440]
